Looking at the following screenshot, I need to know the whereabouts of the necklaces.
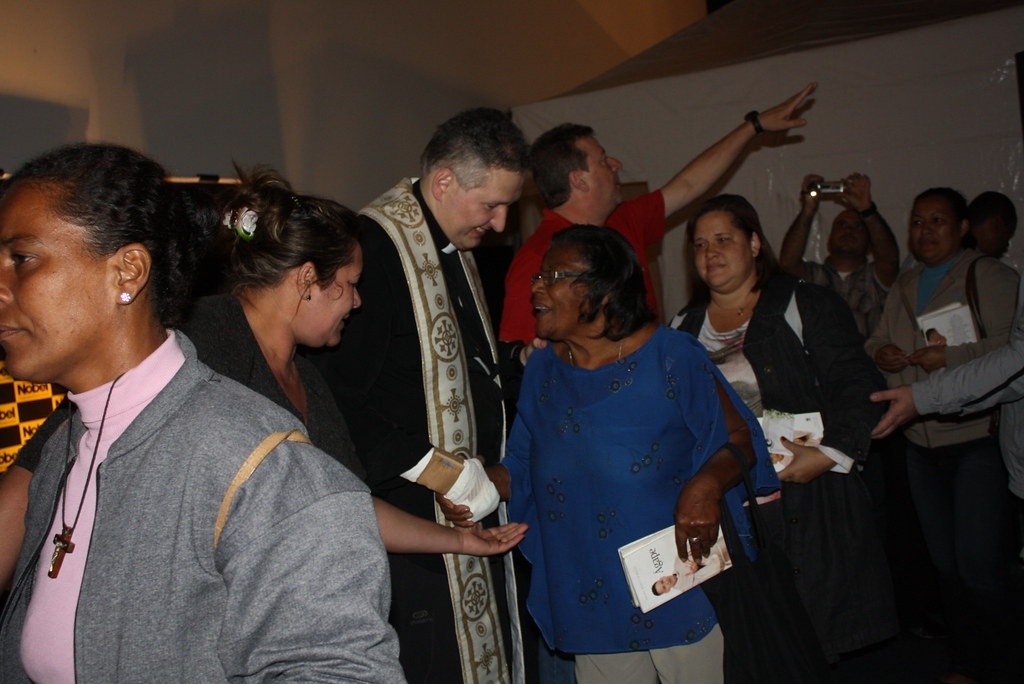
[569,341,623,366]
[712,298,745,316]
[47,373,124,578]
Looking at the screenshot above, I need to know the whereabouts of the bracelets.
[512,345,524,373]
[858,201,876,217]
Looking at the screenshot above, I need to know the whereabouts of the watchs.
[744,110,764,134]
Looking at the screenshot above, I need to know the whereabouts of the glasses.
[530,268,584,287]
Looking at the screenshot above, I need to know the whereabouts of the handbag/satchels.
[710,443,827,684]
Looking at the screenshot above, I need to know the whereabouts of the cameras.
[808,180,844,197]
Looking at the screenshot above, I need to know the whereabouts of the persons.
[667,194,900,684]
[499,80,816,343]
[780,174,945,641]
[969,191,1017,260]
[437,223,780,684]
[869,271,1024,631]
[926,328,947,345]
[0,142,409,684]
[652,537,732,596]
[319,109,546,684]
[185,174,528,555]
[864,187,1024,684]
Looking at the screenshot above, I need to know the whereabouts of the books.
[756,411,824,473]
[916,302,976,347]
[616,522,733,614]
